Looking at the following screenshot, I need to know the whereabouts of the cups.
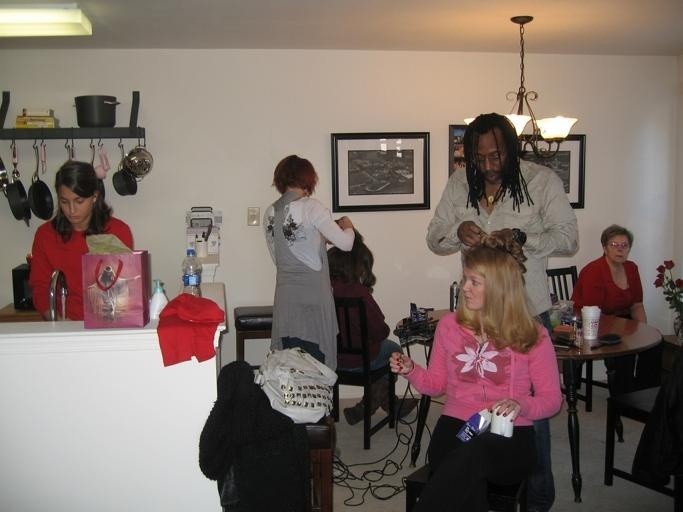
[195,238,208,258]
[580,305,601,340]
[111,164,136,196]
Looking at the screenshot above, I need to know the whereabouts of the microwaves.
[12,264,36,311]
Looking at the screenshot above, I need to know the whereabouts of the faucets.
[48,268,68,319]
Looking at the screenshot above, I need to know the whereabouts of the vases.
[673,318,683,340]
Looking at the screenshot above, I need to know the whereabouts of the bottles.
[180,249,201,300]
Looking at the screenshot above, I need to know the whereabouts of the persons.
[324,240,422,426]
[428,113,581,510]
[27,160,134,323]
[265,155,358,375]
[570,224,663,395]
[388,245,563,509]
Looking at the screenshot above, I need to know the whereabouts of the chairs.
[544,264,594,413]
[332,295,397,451]
[402,310,557,512]
[216,360,336,512]
[603,342,682,512]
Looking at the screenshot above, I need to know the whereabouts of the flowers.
[651,259,683,335]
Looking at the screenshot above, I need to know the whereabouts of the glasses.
[604,242,631,249]
[472,148,507,163]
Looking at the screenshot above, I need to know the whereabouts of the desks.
[658,332,682,387]
[393,307,666,505]
[0,303,45,321]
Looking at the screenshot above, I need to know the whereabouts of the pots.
[117,146,154,183]
[27,175,54,221]
[0,156,8,198]
[72,94,121,127]
[6,172,32,228]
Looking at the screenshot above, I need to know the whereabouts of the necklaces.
[479,181,506,205]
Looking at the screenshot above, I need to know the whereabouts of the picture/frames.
[448,124,471,179]
[329,131,431,214]
[515,133,587,210]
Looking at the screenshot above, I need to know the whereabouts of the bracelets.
[513,229,526,246]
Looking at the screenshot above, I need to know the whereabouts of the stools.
[234,305,272,361]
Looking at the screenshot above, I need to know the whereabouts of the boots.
[343,377,419,425]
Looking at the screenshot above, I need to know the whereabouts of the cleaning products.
[148,278,168,325]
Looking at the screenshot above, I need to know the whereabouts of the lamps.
[0,2,95,38]
[462,15,579,163]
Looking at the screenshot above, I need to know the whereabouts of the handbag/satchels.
[254,348,338,423]
[81,251,149,327]
